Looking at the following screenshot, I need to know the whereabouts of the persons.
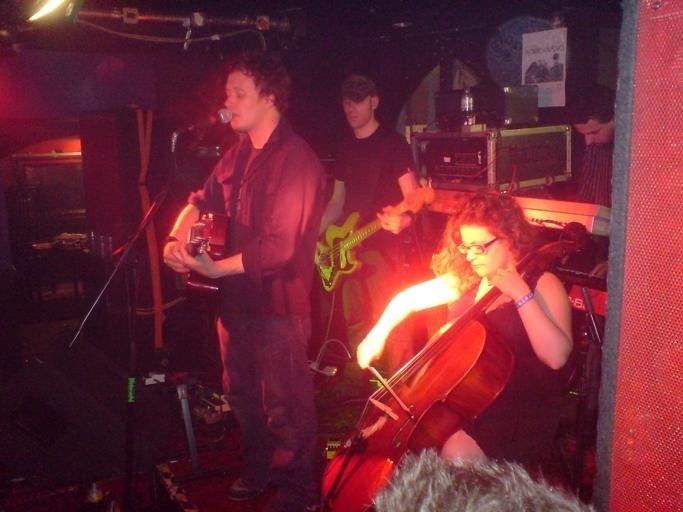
[355,189,577,461]
[161,46,329,512]
[559,95,615,280]
[318,72,423,405]
[369,445,595,512]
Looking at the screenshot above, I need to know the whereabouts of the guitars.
[315,177,435,292]
[177,208,230,314]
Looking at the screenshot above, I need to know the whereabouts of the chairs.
[4,182,79,310]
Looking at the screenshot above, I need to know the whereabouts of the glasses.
[457,238,496,254]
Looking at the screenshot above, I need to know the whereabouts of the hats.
[339,75,376,102]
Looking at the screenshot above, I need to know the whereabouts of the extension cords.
[310,361,337,377]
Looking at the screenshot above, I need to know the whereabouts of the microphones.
[176,108,232,135]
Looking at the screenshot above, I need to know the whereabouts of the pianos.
[554,257,608,317]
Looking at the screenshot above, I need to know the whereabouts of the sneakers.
[230,477,266,501]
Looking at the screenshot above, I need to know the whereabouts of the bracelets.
[403,210,417,224]
[513,291,535,310]
[164,235,180,243]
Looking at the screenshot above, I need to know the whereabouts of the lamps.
[25,0,84,33]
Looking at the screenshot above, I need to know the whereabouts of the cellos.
[321,218,588,512]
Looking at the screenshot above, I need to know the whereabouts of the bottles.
[460,87,476,129]
